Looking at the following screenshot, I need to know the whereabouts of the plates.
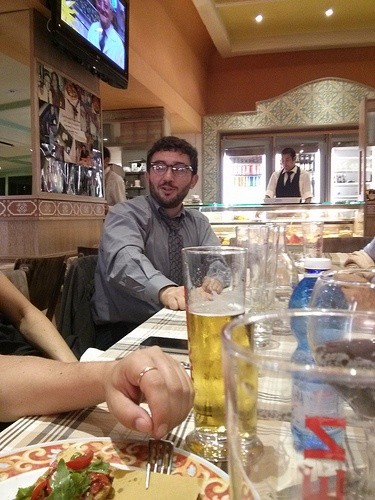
[0,437,260,500]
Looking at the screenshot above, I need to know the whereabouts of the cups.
[181,246,260,467]
[221,309,375,500]
[230,222,325,350]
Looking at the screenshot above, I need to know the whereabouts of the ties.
[285,171,294,188]
[159,212,186,286]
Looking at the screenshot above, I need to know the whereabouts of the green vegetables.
[13,452,112,500]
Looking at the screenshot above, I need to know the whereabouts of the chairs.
[8,242,103,364]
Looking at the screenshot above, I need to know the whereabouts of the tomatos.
[89,473,109,494]
[31,479,53,500]
[65,450,95,469]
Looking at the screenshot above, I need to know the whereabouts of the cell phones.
[140,336,189,354]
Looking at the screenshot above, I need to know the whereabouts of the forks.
[146,435,174,489]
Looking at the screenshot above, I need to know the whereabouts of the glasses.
[150,162,193,176]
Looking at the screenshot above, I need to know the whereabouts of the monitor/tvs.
[48,0,130,89]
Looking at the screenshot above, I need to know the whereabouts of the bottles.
[287,258,349,458]
[296,154,314,172]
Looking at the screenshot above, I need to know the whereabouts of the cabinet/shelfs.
[199,205,364,243]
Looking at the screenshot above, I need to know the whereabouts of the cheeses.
[105,470,203,500]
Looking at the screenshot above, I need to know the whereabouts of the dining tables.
[0,241,374,499]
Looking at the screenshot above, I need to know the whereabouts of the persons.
[93,137,232,350]
[87,0,124,69]
[37,71,64,108]
[80,144,89,163]
[266,147,313,200]
[0,270,195,439]
[344,237,375,284]
[102,146,127,214]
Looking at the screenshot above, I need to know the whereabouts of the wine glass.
[307,268,374,499]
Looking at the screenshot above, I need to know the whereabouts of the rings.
[136,367,158,384]
